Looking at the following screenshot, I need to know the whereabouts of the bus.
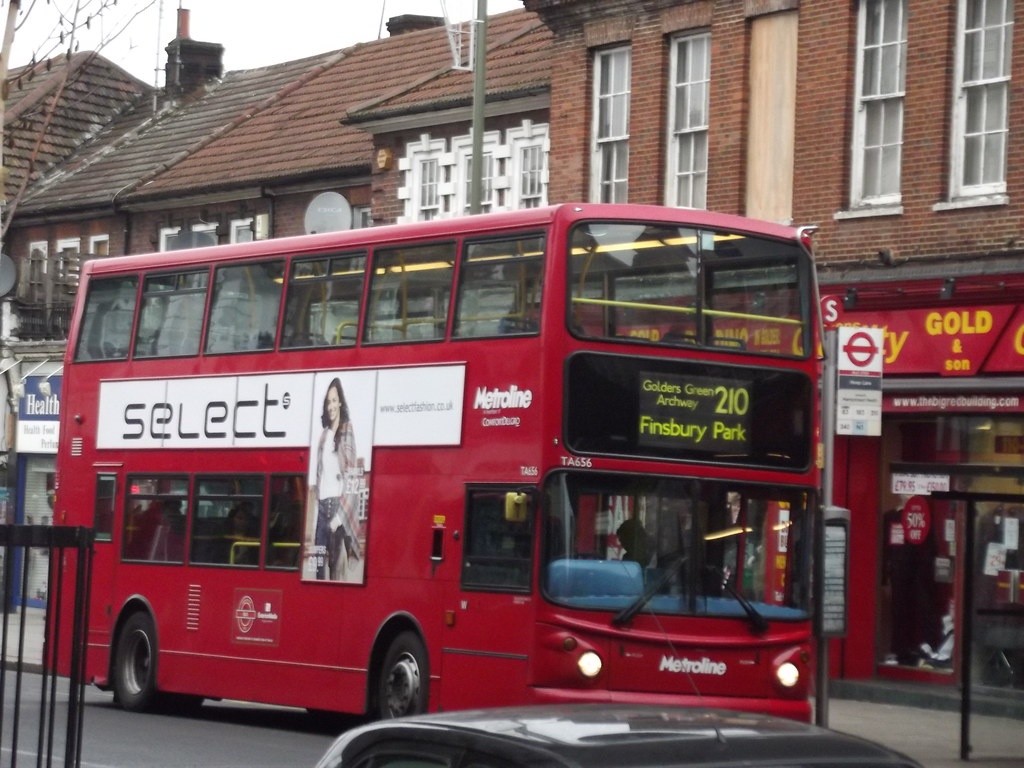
[41,199,829,731]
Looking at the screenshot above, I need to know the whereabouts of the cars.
[312,700,925,768]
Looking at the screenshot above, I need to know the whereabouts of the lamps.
[842,286,905,310]
[938,277,1005,302]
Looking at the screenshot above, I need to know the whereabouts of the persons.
[218,505,259,561]
[310,376,367,580]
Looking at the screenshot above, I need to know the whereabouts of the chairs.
[108,329,311,358]
